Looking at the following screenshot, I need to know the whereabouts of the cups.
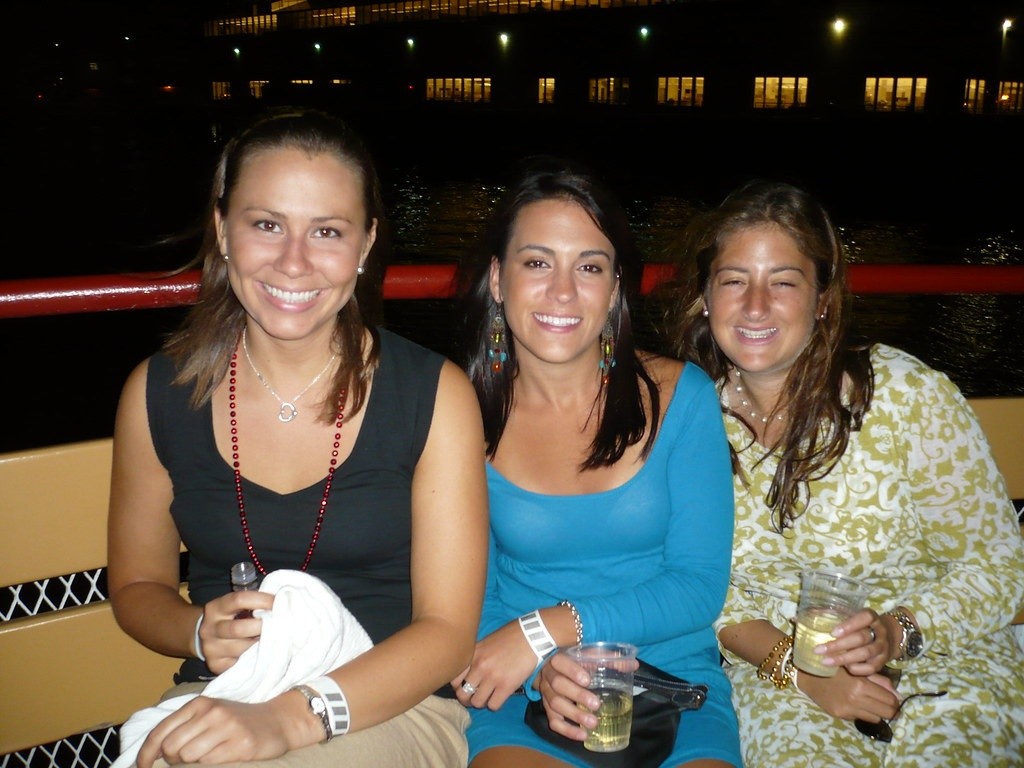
[565,641,638,753]
[790,569,872,678]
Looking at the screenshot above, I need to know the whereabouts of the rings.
[868,625,876,641]
[461,680,474,696]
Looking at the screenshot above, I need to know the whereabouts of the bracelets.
[560,600,584,658]
[518,609,556,661]
[106,110,489,768]
[757,635,799,689]
[195,614,205,661]
[306,676,350,736]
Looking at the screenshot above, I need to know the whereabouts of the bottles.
[230,563,261,620]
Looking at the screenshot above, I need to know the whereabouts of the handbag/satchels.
[525,644,708,768]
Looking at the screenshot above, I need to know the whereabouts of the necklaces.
[243,326,341,421]
[230,348,344,576]
[735,372,783,421]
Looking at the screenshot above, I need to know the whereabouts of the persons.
[449,163,744,768]
[657,178,1024,768]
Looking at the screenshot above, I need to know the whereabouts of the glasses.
[854,691,945,742]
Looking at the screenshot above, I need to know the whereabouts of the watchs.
[885,609,923,659]
[293,685,332,742]
